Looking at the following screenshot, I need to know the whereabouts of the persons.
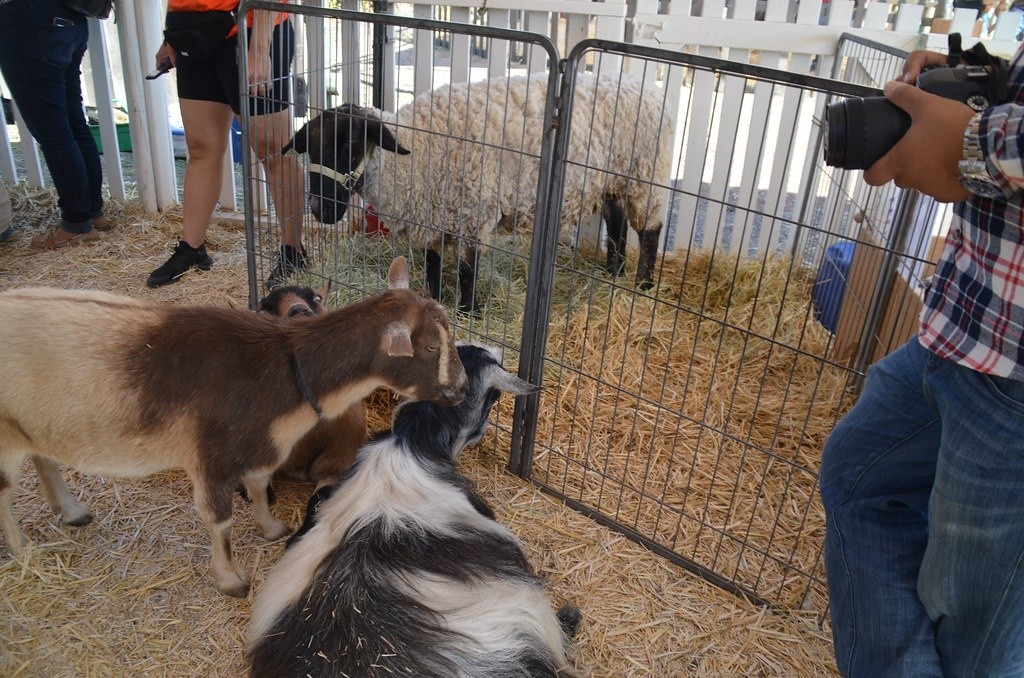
[147,0,304,287]
[0,0,112,249]
[820,37,1024,678]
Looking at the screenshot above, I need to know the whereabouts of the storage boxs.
[84,105,132,153]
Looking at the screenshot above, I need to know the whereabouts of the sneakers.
[266,241,312,289]
[146,240,214,288]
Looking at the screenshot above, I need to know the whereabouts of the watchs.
[958,112,1002,199]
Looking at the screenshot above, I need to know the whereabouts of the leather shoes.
[30,213,110,248]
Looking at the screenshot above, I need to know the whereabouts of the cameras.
[823,60,993,172]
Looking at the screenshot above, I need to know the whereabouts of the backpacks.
[62,0,114,19]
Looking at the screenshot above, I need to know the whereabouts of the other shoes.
[0,227,12,243]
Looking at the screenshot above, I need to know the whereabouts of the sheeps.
[241,343,578,678]
[258,284,371,548]
[0,256,470,594]
[279,72,672,310]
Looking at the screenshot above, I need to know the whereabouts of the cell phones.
[145,61,173,80]
